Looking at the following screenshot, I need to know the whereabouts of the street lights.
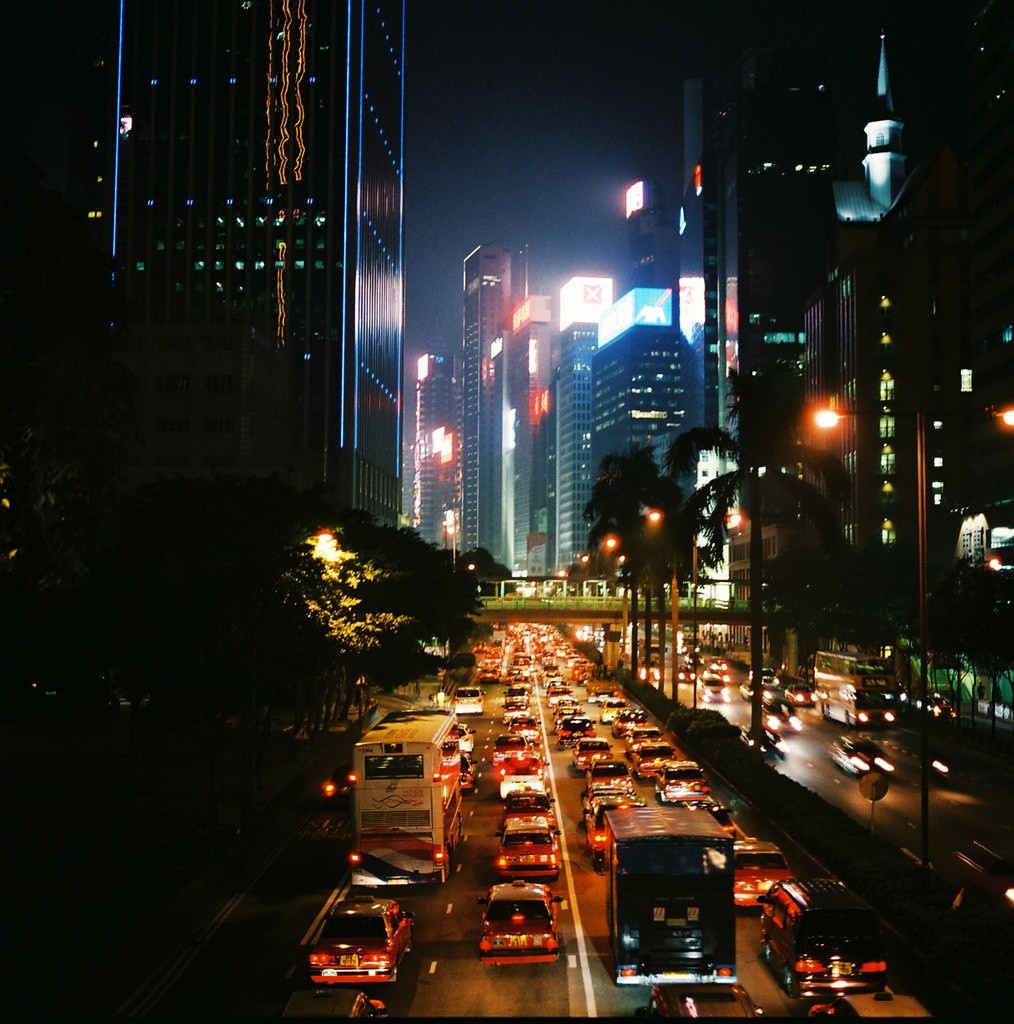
[640,507,741,709]
[812,405,1014,869]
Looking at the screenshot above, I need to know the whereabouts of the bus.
[814,650,893,694]
[606,809,737,987]
[353,708,468,887]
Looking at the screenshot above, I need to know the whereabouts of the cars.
[479,877,563,968]
[675,632,731,702]
[739,667,820,716]
[819,681,897,727]
[829,734,896,775]
[916,693,957,720]
[646,981,770,1023]
[732,838,931,1024]
[446,622,683,804]
[493,805,561,873]
[582,790,632,856]
[639,638,673,681]
[653,756,716,809]
[308,896,418,988]
